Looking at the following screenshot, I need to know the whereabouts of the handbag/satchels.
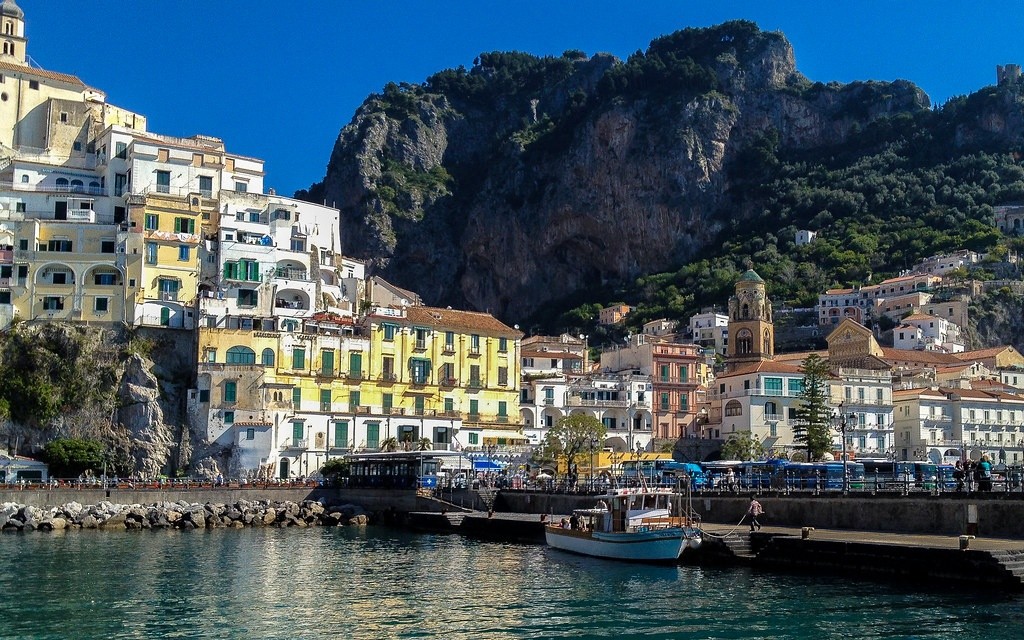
[952,470,965,479]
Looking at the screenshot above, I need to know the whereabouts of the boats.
[544,454,704,566]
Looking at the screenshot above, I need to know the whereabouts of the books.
[689,467,736,491]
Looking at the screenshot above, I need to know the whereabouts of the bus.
[622,458,959,494]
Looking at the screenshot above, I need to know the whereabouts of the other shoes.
[758,524,761,530]
[750,529,755,531]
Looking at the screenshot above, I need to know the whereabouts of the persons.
[747,494,763,532]
[654,473,662,492]
[215,472,222,487]
[558,512,590,534]
[594,499,608,510]
[952,455,994,493]
[572,473,578,491]
